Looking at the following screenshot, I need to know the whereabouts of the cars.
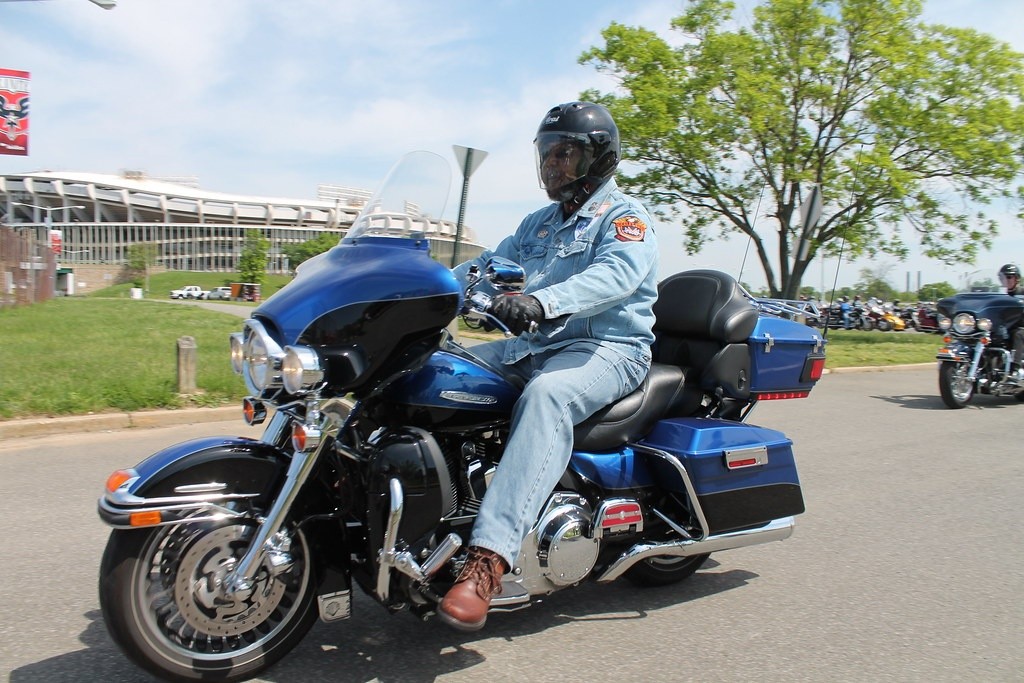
[193,291,212,300]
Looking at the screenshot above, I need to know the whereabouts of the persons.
[758,293,938,329]
[435,99,660,631]
[997,264,1024,387]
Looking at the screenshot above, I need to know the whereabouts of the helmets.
[533,101,623,202]
[998,263,1021,279]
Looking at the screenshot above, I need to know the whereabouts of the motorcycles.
[95,142,870,682]
[933,290,1024,409]
[754,290,941,335]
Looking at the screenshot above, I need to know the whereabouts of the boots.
[436,546,506,632]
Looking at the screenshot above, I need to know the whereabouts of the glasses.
[541,146,583,159]
[1006,275,1015,279]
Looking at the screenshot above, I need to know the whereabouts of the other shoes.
[1009,370,1020,376]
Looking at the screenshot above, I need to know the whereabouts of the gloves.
[483,295,544,336]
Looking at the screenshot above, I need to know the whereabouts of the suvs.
[208,286,232,300]
[169,286,202,299]
